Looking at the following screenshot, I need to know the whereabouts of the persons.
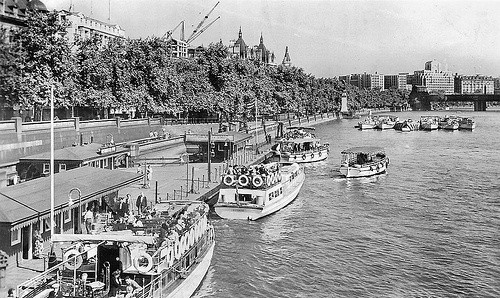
[217,120,320,159]
[147,166,152,186]
[353,151,373,166]
[78,194,207,298]
[227,162,281,204]
[137,164,141,183]
[149,129,172,141]
[357,112,476,134]
[32,226,44,258]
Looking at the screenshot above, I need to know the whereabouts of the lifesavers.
[252,176,264,187]
[62,248,83,271]
[132,253,153,273]
[238,175,249,187]
[223,175,233,186]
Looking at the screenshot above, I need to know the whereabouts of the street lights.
[222,138,231,174]
[103,132,118,146]
[66,187,87,235]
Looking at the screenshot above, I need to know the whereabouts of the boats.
[13,197,216,297]
[355,114,387,130]
[438,118,459,130]
[336,147,387,177]
[457,117,476,130]
[394,117,420,132]
[214,158,305,223]
[266,122,330,162]
[376,116,399,131]
[420,114,441,131]
